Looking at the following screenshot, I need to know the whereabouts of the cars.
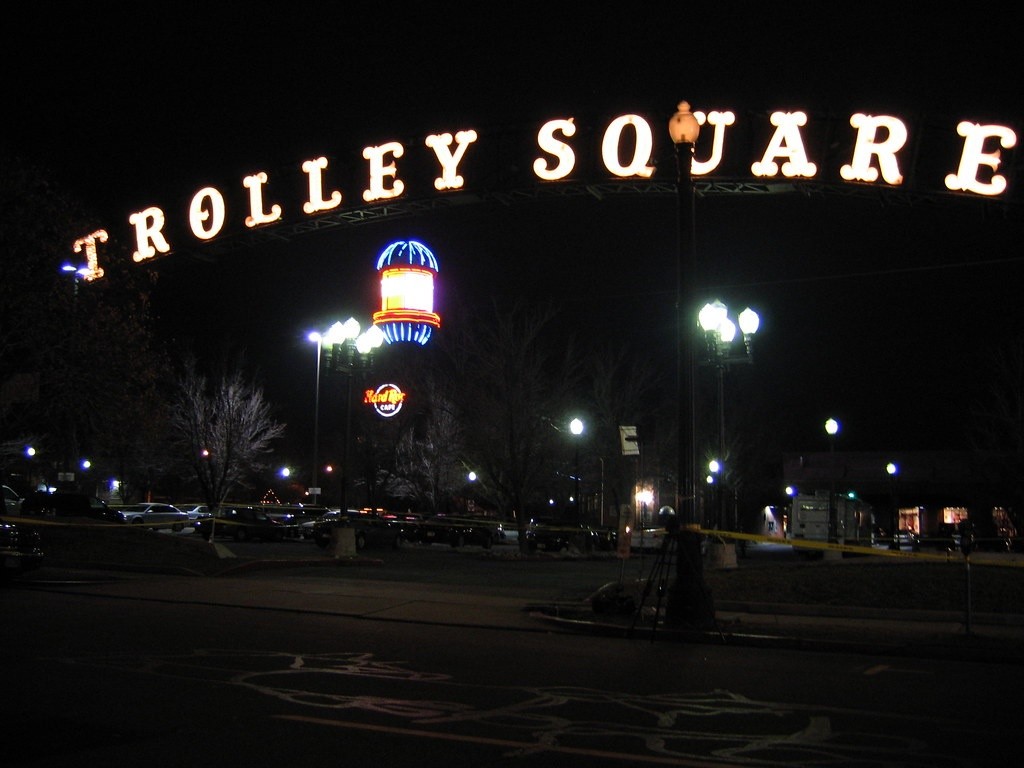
[19,482,124,524]
[302,509,361,540]
[194,506,284,542]
[121,503,189,523]
[185,506,212,520]
[314,514,405,551]
[3,485,25,516]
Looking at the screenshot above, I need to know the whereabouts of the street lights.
[706,475,714,529]
[340,316,386,517]
[825,417,839,544]
[27,446,36,482]
[886,463,896,550]
[83,461,98,497]
[305,327,333,504]
[567,417,588,529]
[784,487,793,538]
[694,299,761,531]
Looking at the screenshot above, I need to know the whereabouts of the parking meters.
[958,519,976,633]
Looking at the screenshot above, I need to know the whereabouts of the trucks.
[791,489,875,548]
